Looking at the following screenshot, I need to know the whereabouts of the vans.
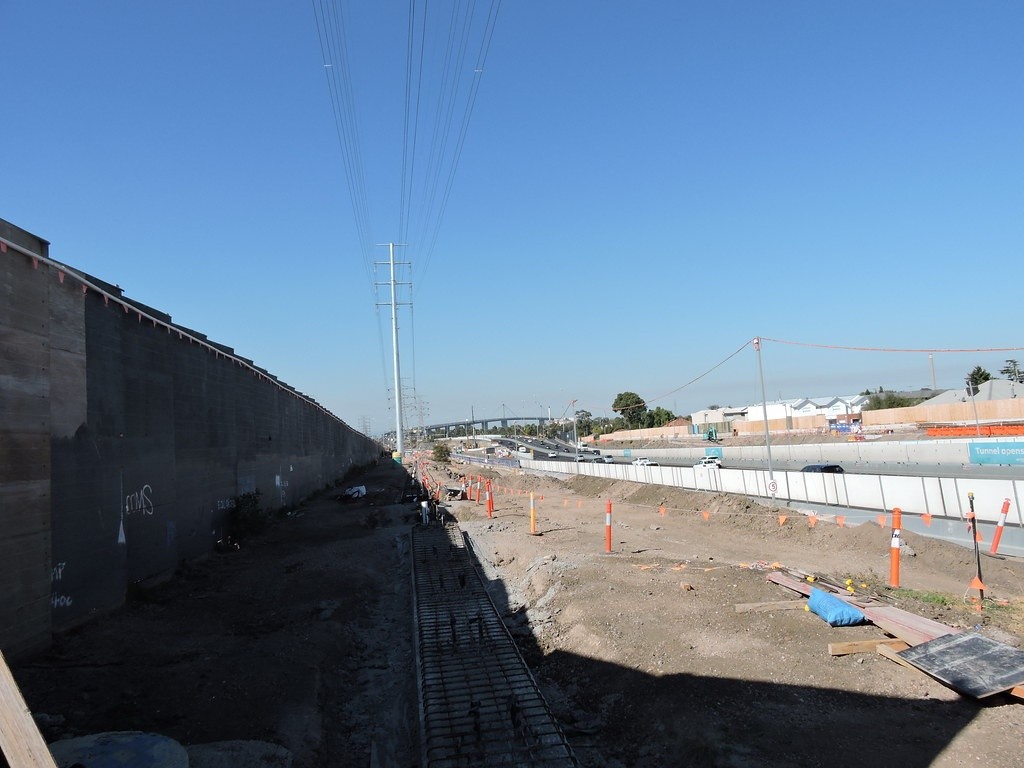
[603,455,614,463]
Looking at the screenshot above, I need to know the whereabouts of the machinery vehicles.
[703,427,717,442]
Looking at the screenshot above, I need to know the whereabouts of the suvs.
[693,460,719,468]
[698,456,722,467]
[800,464,845,474]
[574,455,585,462]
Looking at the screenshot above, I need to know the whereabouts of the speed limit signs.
[769,482,777,492]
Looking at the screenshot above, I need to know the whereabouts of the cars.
[499,435,570,453]
[590,458,604,464]
[548,452,556,458]
[638,461,660,467]
[631,457,650,465]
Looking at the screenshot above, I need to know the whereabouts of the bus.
[580,443,588,451]
